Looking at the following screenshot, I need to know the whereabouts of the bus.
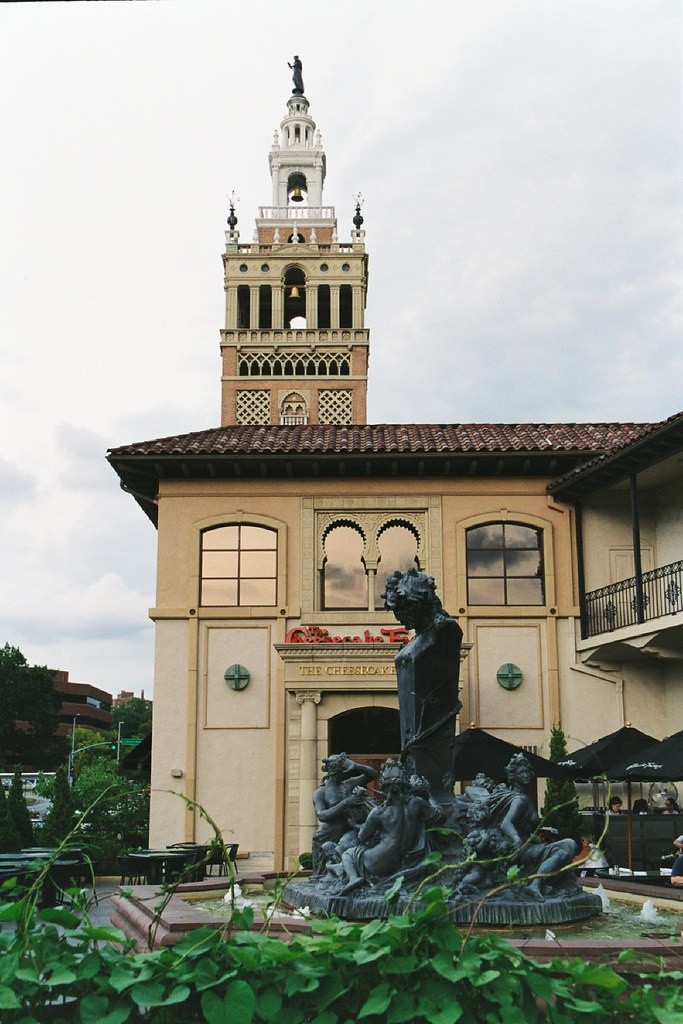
[0,772,57,790]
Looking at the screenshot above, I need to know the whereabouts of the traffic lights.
[110,735,118,751]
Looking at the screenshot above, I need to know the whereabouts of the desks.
[175,844,230,848]
[597,870,671,884]
[647,858,675,870]
[0,848,81,909]
[128,853,187,885]
[144,849,196,852]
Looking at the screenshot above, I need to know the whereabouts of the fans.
[648,782,678,807]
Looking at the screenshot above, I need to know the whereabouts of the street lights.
[117,721,125,765]
[69,714,81,787]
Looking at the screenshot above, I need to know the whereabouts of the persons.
[673,835,683,858]
[312,568,579,903]
[661,798,679,814]
[670,856,683,887]
[291,55,304,93]
[570,833,609,868]
[606,796,624,815]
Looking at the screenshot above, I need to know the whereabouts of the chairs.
[0,861,100,910]
[117,841,239,886]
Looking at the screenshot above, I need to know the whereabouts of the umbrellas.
[441,720,683,869]
